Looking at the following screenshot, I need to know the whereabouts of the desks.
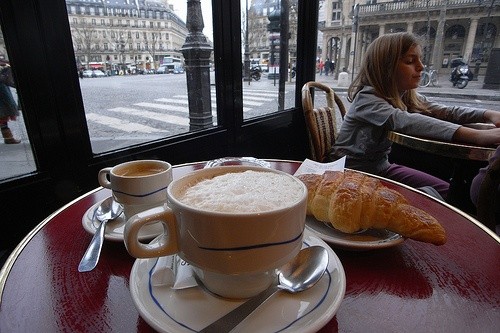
[387,123,500,189]
[0,158,500,333]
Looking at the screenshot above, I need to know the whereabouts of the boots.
[1,128,21,144]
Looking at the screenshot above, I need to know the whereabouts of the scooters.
[249,69,261,80]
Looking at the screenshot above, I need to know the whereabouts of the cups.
[123,165,309,301]
[98,160,172,226]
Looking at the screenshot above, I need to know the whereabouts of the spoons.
[77,195,120,272]
[199,245,329,333]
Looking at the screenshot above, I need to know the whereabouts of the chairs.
[302,81,346,162]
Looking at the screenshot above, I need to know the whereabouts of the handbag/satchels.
[1,65,16,88]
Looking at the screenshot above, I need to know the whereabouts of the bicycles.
[417,63,439,88]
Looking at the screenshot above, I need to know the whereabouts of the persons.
[325,58,331,75]
[0,26,22,144]
[319,61,324,76]
[323,32,500,238]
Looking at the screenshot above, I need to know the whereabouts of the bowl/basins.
[203,156,271,169]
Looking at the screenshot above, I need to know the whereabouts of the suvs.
[155,66,168,74]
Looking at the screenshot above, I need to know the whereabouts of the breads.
[292,171,447,246]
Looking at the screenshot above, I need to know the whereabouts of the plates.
[304,217,409,248]
[129,229,346,333]
[82,196,164,239]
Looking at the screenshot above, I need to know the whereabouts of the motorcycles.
[448,51,474,89]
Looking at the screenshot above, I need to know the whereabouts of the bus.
[163,56,182,72]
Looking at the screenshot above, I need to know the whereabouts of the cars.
[81,69,108,78]
